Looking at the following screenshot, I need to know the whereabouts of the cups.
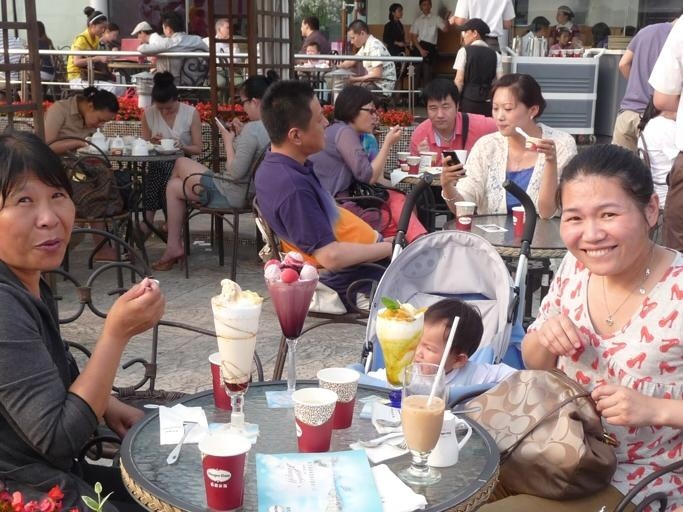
[197,433,250,511]
[452,199,477,233]
[79,128,159,160]
[316,366,361,430]
[510,206,526,236]
[158,139,180,149]
[550,47,584,59]
[397,148,467,176]
[290,386,340,454]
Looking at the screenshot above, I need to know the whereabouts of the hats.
[458,18,491,34]
[557,6,576,16]
[130,21,153,36]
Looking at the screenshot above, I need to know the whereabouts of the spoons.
[140,277,160,292]
[375,418,400,427]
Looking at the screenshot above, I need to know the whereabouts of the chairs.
[34,222,267,479]
[614,458,681,511]
[43,114,396,381]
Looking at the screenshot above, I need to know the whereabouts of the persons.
[256,79,394,312]
[384,0,517,118]
[612,13,683,251]
[0,132,165,511]
[440,72,577,219]
[368,300,519,387]
[523,4,611,61]
[339,20,397,104]
[475,144,683,512]
[412,80,498,167]
[0,8,243,85]
[44,69,278,272]
[310,87,428,244]
[298,15,330,63]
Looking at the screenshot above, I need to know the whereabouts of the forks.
[166,420,199,464]
[398,439,407,449]
[360,434,406,450]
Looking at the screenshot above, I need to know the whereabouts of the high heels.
[152,254,185,272]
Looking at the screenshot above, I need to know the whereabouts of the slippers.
[92,251,131,264]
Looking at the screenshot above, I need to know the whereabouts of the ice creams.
[262,251,320,340]
[375,295,428,385]
[209,277,264,397]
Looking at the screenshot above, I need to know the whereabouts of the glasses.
[360,108,377,115]
[239,98,252,105]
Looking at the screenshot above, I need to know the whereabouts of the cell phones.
[214,117,229,130]
[441,150,465,177]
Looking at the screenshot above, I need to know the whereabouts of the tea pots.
[418,408,472,469]
[508,34,548,57]
[327,48,340,70]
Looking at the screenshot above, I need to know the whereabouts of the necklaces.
[602,243,655,324]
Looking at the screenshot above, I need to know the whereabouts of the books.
[256,449,381,512]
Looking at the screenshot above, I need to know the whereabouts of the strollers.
[345,175,536,409]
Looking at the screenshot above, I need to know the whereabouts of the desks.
[436,211,580,266]
[116,378,506,512]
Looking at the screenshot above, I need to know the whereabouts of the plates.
[155,147,179,155]
[296,61,329,70]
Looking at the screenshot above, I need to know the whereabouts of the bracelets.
[441,187,458,201]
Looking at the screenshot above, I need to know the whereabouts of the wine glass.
[211,295,263,437]
[400,362,444,488]
[264,273,320,406]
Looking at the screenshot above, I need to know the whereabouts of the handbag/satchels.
[68,166,124,217]
[348,177,389,208]
[79,60,111,81]
[464,368,615,501]
